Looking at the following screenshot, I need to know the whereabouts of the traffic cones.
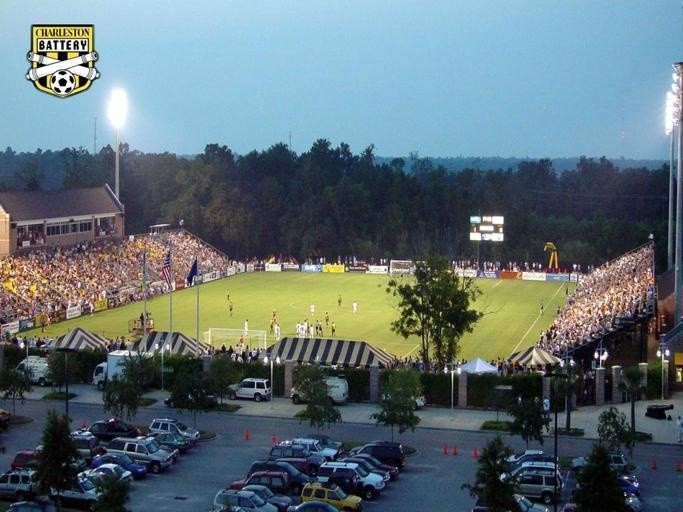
[443,443,448,455]
[244,429,250,441]
[453,444,458,455]
[676,462,681,472]
[652,458,657,469]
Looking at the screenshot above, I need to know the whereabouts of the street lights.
[655,340,671,401]
[263,352,281,400]
[560,351,574,429]
[110,89,126,199]
[442,360,462,409]
[670,60,683,328]
[543,372,568,512]
[155,337,169,392]
[591,340,608,367]
[662,87,675,270]
[19,339,31,392]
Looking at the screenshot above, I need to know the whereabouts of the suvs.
[213,434,406,512]
[226,378,271,402]
[0,417,201,512]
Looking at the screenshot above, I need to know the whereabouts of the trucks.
[10,356,55,386]
[91,350,154,392]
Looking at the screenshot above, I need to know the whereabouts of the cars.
[0,408,11,429]
[471,447,643,511]
[382,388,425,410]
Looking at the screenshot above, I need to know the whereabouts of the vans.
[290,374,349,404]
